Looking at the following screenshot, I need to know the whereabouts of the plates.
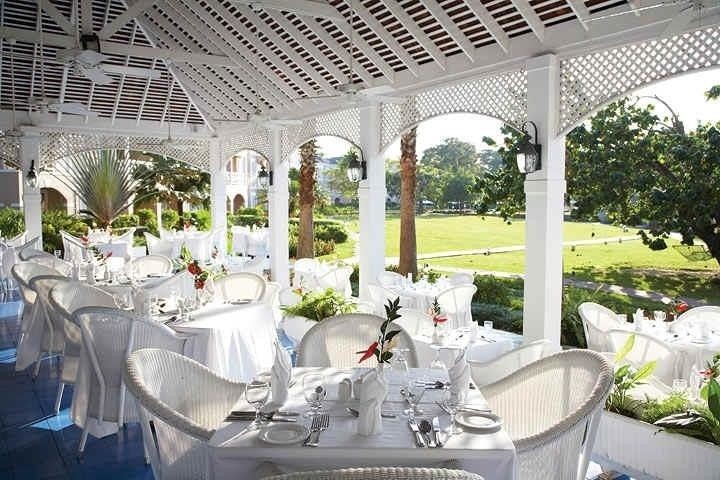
[259,423,311,444]
[455,410,504,429]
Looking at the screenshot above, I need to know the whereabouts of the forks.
[304,417,321,446]
[311,415,329,447]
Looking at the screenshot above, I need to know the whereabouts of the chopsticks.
[224,411,300,422]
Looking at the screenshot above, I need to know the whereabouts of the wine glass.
[388,348,413,387]
[484,321,492,336]
[245,380,270,426]
[303,374,328,419]
[673,379,688,390]
[427,346,448,381]
[113,291,127,310]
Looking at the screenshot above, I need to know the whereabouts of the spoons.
[419,420,436,448]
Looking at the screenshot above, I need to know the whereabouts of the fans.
[56,0,162,89]
[212,7,302,131]
[137,60,202,150]
[0,38,50,142]
[14,0,90,115]
[295,0,406,105]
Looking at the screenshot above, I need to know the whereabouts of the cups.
[654,310,666,322]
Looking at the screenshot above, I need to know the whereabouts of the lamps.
[512,120,543,174]
[258,160,273,187]
[346,141,367,187]
[24,159,39,190]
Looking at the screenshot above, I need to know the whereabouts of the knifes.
[433,416,444,446]
[408,421,425,447]
